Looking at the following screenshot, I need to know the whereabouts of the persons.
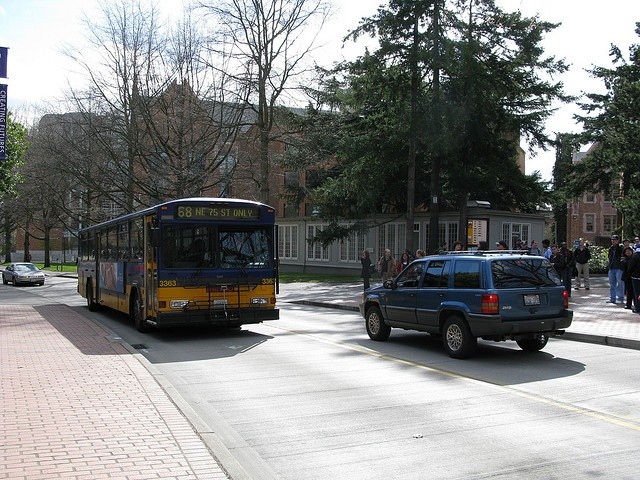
[560,241,573,298]
[377,249,395,283]
[574,238,591,291]
[550,246,564,279]
[433,239,542,260]
[415,250,425,260]
[542,240,552,263]
[606,234,640,315]
[396,253,413,280]
[360,250,374,291]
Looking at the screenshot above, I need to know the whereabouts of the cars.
[2,262,45,286]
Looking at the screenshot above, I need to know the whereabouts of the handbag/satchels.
[566,262,578,279]
[378,270,383,278]
[369,264,376,273]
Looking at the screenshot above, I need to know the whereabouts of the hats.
[610,235,620,240]
[495,241,506,246]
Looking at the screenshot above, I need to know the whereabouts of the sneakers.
[616,300,623,303]
[574,288,579,292]
[606,300,614,302]
[633,308,639,313]
[625,305,631,309]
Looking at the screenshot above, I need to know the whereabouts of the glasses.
[497,244,501,247]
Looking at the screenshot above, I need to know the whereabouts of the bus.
[76,197,280,333]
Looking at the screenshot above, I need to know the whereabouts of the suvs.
[359,250,574,359]
